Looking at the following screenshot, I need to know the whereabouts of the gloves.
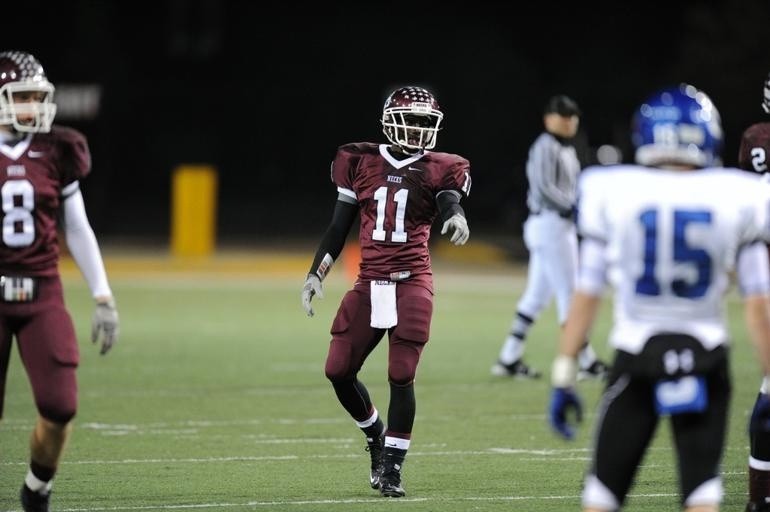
[546,386,585,443]
[300,274,327,319]
[90,301,125,357]
[439,212,472,248]
[746,389,770,437]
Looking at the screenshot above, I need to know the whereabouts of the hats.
[542,93,583,119]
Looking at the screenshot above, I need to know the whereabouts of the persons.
[489,92,610,382]
[301,84,474,497]
[549,80,770,511]
[1,50,119,511]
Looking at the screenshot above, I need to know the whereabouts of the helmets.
[629,82,729,168]
[762,76,770,114]
[381,83,445,133]
[0,48,56,104]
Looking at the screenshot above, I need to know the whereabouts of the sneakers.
[576,358,616,384]
[364,424,389,489]
[379,452,407,498]
[18,480,53,512]
[490,355,545,381]
